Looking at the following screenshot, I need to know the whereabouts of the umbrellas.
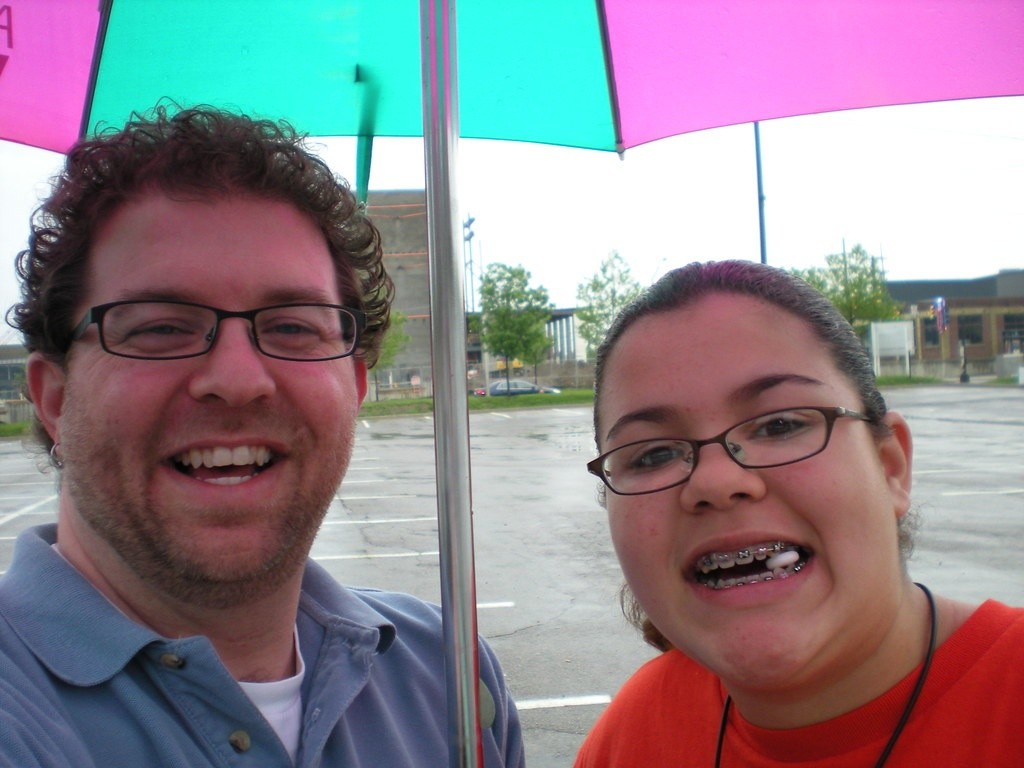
[0,1,1024,767]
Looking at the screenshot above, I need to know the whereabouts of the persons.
[575,261,1024,768]
[0,104,525,767]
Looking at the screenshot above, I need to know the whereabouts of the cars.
[473,379,562,396]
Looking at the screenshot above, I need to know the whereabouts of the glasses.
[586,406,872,495]
[72,298,365,362]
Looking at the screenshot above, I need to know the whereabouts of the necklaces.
[713,582,938,768]
